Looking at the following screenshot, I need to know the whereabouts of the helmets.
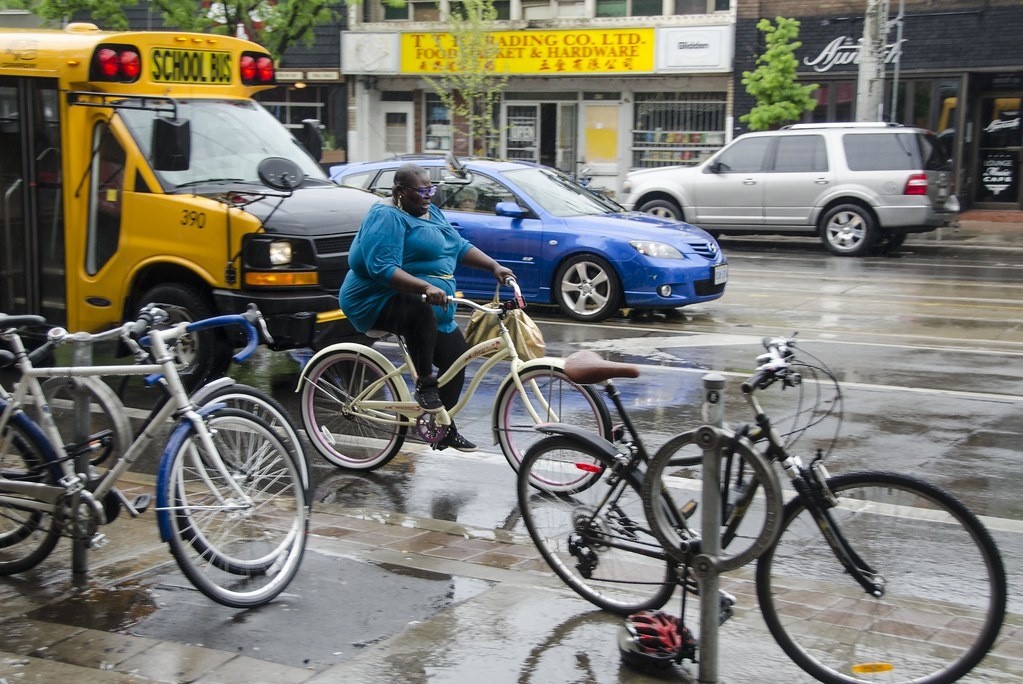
[619,609,695,667]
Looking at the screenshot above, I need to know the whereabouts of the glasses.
[398,182,438,198]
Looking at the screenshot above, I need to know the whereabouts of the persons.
[339,164,516,453]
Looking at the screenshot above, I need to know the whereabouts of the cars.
[326,154,729,324]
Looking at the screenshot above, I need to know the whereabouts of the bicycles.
[515,331,1007,684]
[0,303,312,609]
[292,275,615,497]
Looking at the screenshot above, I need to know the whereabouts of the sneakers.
[411,377,443,413]
[430,417,479,452]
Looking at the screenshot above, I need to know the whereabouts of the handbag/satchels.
[464,281,546,362]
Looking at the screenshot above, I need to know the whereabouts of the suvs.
[615,118,961,257]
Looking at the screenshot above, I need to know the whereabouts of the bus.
[0,18,399,398]
